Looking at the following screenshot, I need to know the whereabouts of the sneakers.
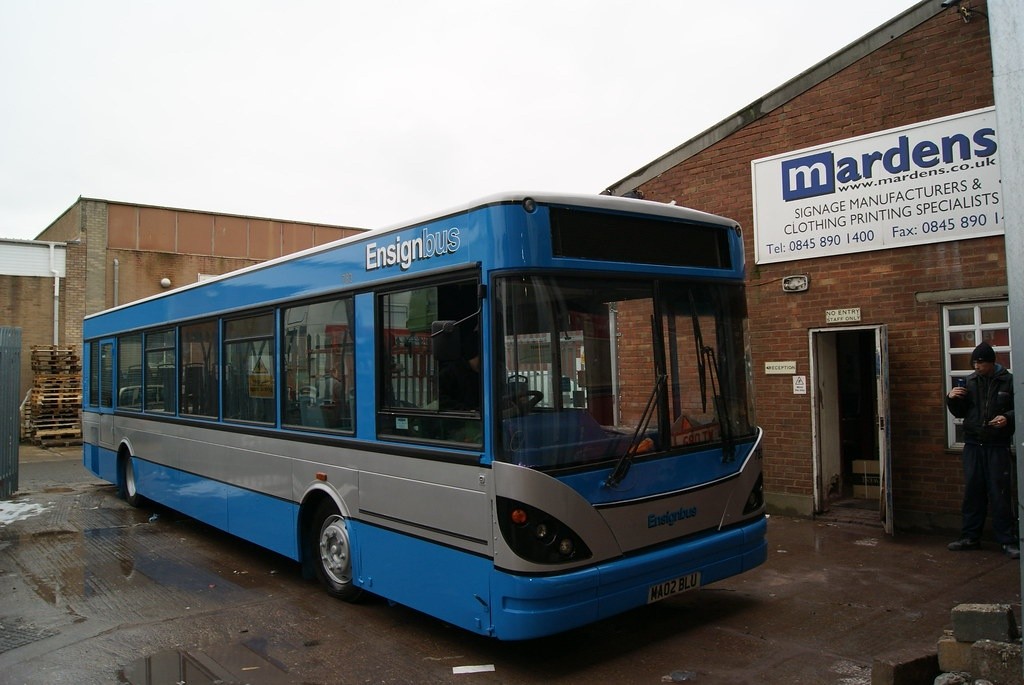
[999,542,1021,558]
[947,536,982,550]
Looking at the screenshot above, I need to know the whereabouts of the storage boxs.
[852,460,880,499]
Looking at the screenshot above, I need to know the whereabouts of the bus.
[82,190,770,642]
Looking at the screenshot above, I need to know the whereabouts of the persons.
[946,340,1020,559]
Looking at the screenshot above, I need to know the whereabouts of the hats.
[970,341,995,363]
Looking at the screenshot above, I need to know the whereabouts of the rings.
[997,421,1001,424]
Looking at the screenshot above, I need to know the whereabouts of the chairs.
[107,364,356,431]
[507,374,528,414]
